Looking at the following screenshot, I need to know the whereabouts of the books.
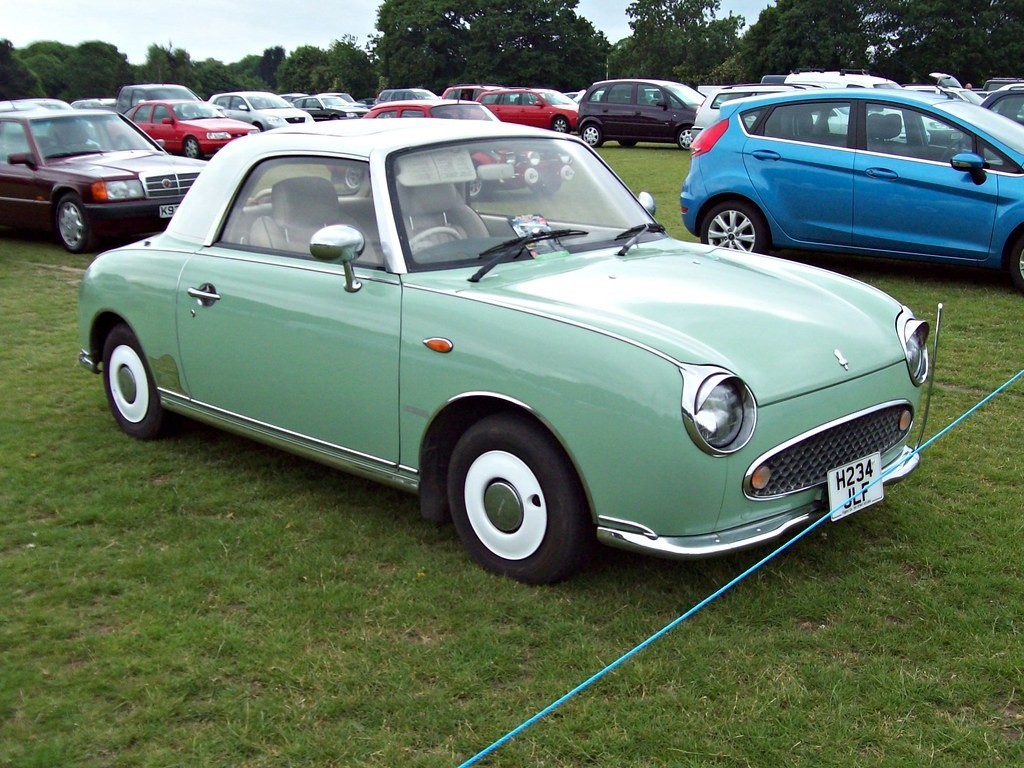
[508,213,569,261]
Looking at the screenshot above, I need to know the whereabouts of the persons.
[965,83,972,90]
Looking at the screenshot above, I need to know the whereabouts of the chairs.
[251,177,379,263]
[502,96,531,106]
[639,89,662,105]
[394,179,489,256]
[866,113,927,159]
[774,109,834,146]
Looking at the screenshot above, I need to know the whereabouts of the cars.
[329,99,573,203]
[78,120,930,584]
[0,110,213,253]
[679,84,1024,287]
[576,77,720,150]
[0,81,579,158]
[747,69,1024,140]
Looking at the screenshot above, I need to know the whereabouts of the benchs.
[223,194,382,265]
